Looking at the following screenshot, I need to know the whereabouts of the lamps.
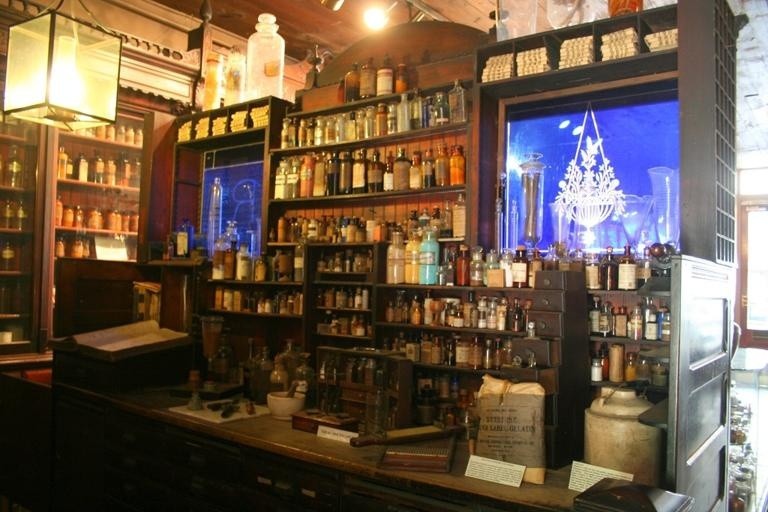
[0,0,126,134]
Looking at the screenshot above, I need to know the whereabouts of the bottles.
[343,50,408,104]
[164,224,672,428]
[0,276,33,314]
[0,111,144,273]
[269,78,474,241]
[200,11,285,111]
[177,175,262,257]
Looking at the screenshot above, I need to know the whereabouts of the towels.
[479,24,678,86]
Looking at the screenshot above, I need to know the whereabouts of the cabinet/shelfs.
[269,73,468,249]
[676,1,751,275]
[307,240,375,438]
[0,75,157,367]
[180,232,251,401]
[588,284,665,400]
[473,4,684,90]
[376,259,587,469]
[251,242,310,413]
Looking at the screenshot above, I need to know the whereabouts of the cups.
[8,324,24,341]
[0,331,12,342]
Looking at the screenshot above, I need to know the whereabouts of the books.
[573,476,695,512]
[47,317,190,363]
